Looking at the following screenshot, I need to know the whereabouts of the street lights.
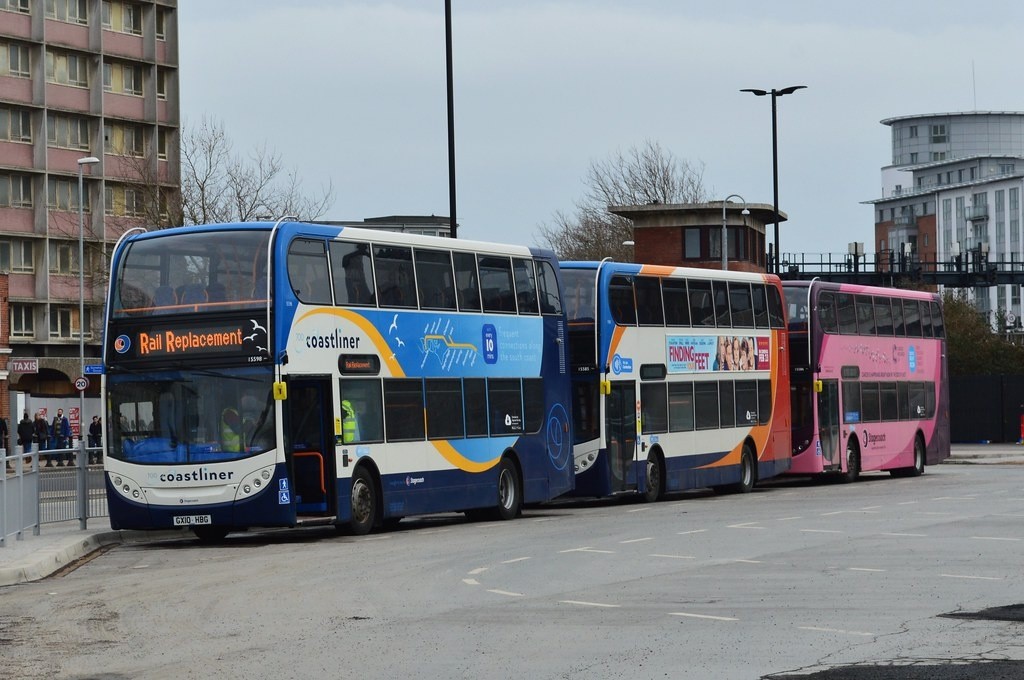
[76,155,102,532]
[739,85,808,280]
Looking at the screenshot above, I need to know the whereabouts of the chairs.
[151,283,542,316]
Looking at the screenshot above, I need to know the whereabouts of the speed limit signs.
[74,377,88,391]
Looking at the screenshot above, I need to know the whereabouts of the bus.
[92,217,580,544]
[555,255,794,508]
[777,277,952,486]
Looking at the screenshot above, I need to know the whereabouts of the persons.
[342,400,361,442]
[0,409,70,469]
[713,336,758,371]
[89,413,129,465]
[221,392,257,453]
